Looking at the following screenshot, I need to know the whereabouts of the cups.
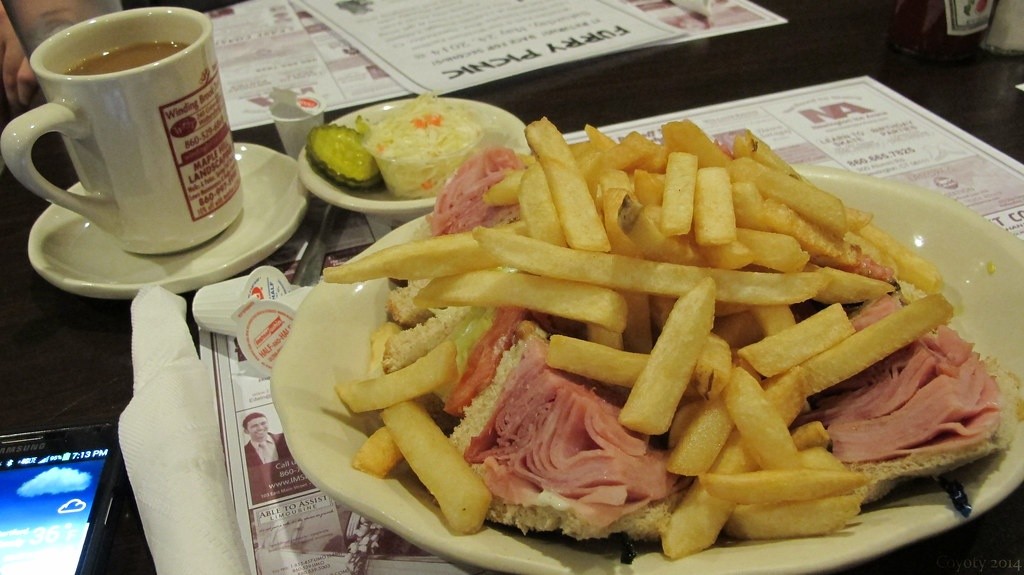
[889,0,994,62]
[0,6,241,256]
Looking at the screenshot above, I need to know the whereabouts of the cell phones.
[0,423,127,575]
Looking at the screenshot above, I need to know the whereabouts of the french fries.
[322,114,953,559]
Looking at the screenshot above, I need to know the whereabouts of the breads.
[378,234,1022,537]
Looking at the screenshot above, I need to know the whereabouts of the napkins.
[119,285,253,575]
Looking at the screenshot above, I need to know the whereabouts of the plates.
[298,98,532,219]
[272,165,1024,575]
[27,142,307,299]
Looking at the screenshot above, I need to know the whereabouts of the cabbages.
[368,90,480,201]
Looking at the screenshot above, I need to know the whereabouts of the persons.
[242,413,295,468]
